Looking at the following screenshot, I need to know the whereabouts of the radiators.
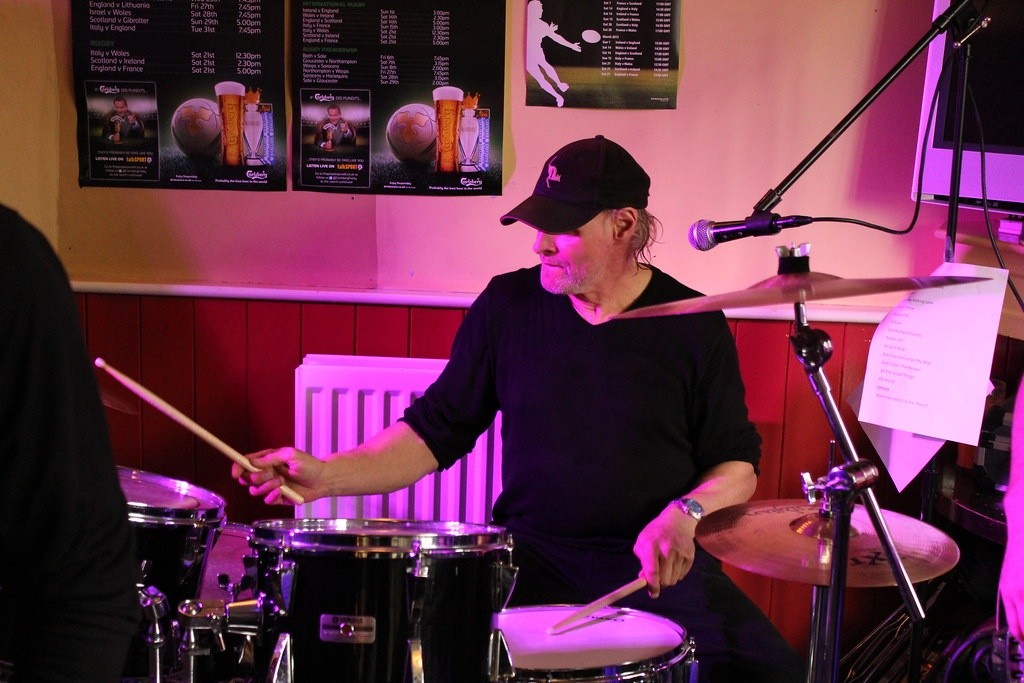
[294,354,504,525]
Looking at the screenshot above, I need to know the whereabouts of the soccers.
[171,97,223,160]
[385,103,438,165]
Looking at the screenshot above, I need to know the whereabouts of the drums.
[175,596,260,682]
[495,600,700,683]
[238,517,519,683]
[113,459,225,683]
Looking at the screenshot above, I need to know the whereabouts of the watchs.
[668,497,703,522]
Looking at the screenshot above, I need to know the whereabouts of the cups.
[476,109,490,172]
[214,82,245,166]
[260,103,276,165]
[432,86,464,173]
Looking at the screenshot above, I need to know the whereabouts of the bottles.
[459,109,480,172]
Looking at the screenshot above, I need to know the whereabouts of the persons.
[0,201,154,682]
[103,97,145,142]
[313,106,356,150]
[228,134,764,683]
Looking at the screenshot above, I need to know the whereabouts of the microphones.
[688,215,812,251]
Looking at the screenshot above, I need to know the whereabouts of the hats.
[500,135,650,234]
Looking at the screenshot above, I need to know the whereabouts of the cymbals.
[606,271,993,319]
[695,497,961,589]
